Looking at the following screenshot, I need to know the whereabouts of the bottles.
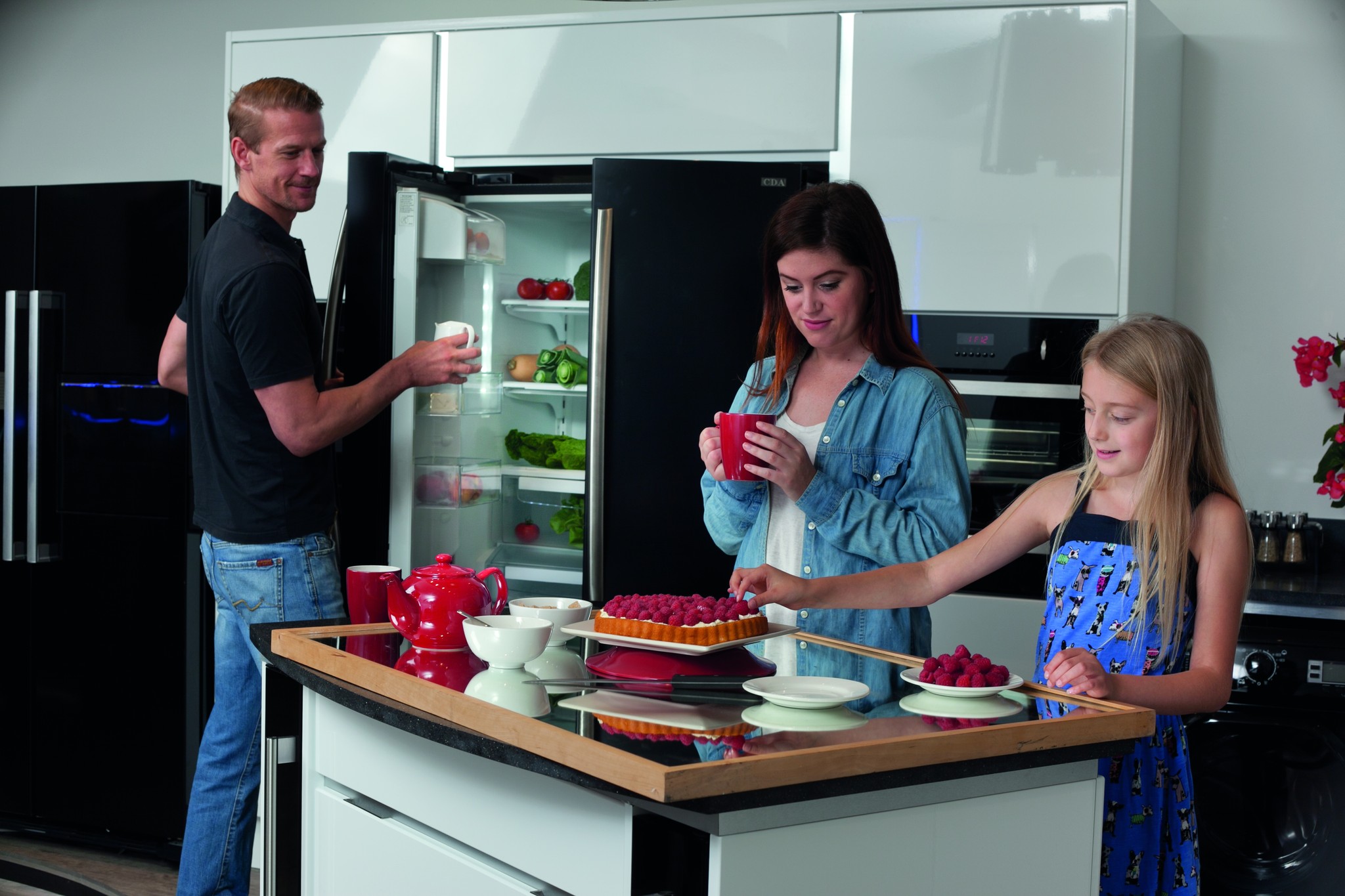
[1244,510,1310,565]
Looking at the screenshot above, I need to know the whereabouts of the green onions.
[532,347,588,389]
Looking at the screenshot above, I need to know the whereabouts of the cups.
[347,565,402,624]
[719,413,777,481]
[346,633,394,669]
[434,320,475,364]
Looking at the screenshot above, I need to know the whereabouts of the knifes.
[524,681,763,706]
[523,674,763,688]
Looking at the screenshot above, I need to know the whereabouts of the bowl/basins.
[509,598,593,647]
[524,645,590,694]
[464,667,551,718]
[462,615,554,669]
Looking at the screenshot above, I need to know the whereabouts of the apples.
[415,471,483,503]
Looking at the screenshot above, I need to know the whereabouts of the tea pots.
[394,646,489,694]
[379,553,508,651]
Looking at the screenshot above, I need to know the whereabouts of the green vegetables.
[505,429,586,471]
[574,260,590,300]
[550,493,584,550]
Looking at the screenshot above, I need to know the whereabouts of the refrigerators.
[0,181,222,833]
[317,151,830,598]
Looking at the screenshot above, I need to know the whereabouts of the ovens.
[901,313,1100,598]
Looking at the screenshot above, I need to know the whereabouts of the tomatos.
[468,228,490,255]
[515,516,541,543]
[517,278,574,300]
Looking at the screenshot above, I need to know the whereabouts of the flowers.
[1290,333,1345,509]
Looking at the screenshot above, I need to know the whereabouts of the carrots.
[507,354,540,382]
[553,345,581,354]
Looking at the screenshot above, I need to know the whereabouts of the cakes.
[591,713,760,748]
[594,594,770,647]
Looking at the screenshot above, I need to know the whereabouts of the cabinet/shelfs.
[438,14,842,171]
[848,0,1182,322]
[221,24,439,307]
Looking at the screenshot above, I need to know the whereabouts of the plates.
[899,690,1023,718]
[741,701,869,732]
[558,689,745,731]
[560,619,800,656]
[900,668,1024,698]
[742,676,870,709]
[586,645,777,682]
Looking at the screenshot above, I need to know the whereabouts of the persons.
[156,73,483,896]
[698,183,971,661]
[694,635,1105,763]
[728,315,1254,896]
